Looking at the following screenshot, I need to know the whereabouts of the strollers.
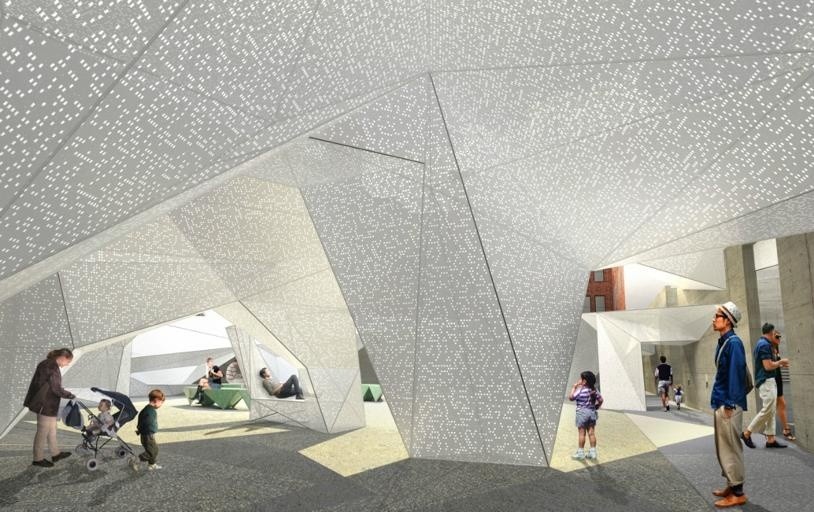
[72,386,139,471]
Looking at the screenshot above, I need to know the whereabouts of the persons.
[131,390,162,471]
[740,323,790,448]
[654,356,673,412]
[188,357,223,404]
[770,331,796,441]
[75,399,114,451]
[568,371,604,461]
[671,385,684,411]
[709,301,748,507]
[259,368,305,399]
[22,348,76,467]
